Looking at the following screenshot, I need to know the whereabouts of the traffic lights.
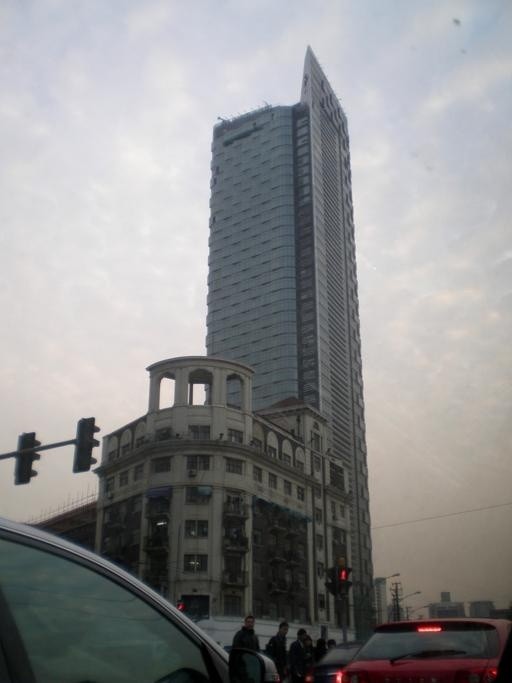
[14,432,42,484]
[73,419,100,473]
[325,566,353,597]
[176,600,183,612]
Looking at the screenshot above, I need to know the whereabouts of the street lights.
[356,574,432,638]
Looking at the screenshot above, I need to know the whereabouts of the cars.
[306,618,511,683]
[1,518,279,683]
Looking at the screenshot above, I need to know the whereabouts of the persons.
[232,616,262,654]
[263,622,336,683]
